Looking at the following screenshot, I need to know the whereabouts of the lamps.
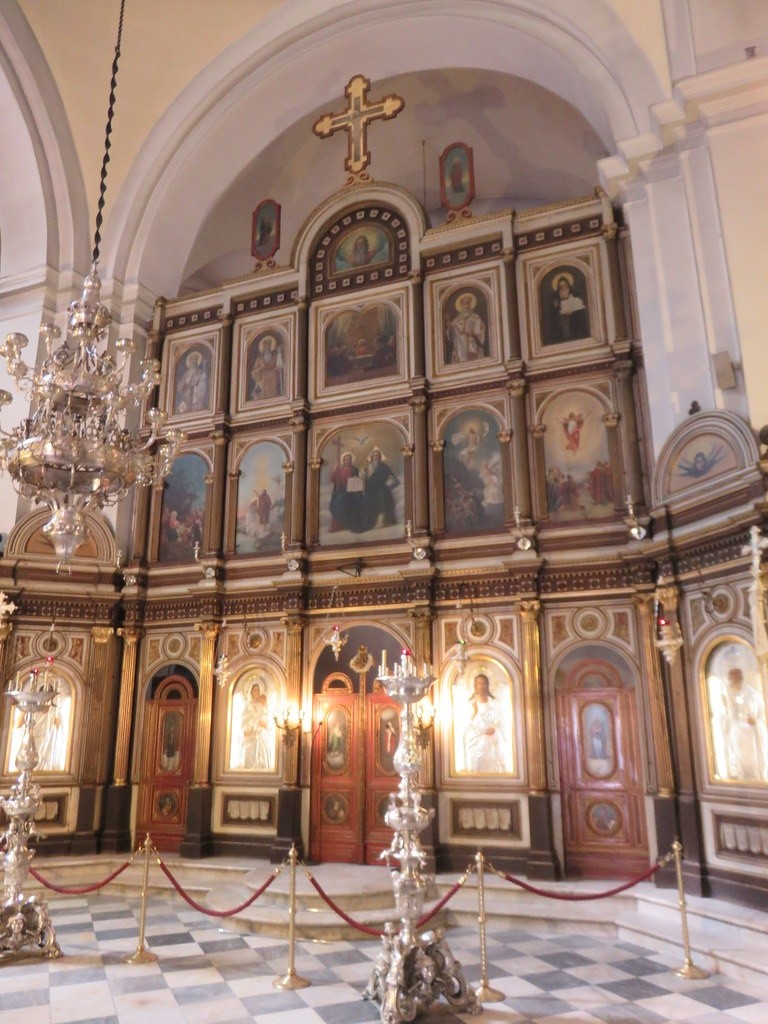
[404,704,436,751]
[653,575,684,667]
[272,709,305,748]
[0,0,189,579]
[208,617,233,690]
[320,585,350,663]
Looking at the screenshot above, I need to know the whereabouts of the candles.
[627,493,631,501]
[195,539,199,547]
[377,648,431,680]
[515,506,519,512]
[281,530,285,537]
[118,550,121,555]
[8,662,59,694]
[408,520,411,526]
